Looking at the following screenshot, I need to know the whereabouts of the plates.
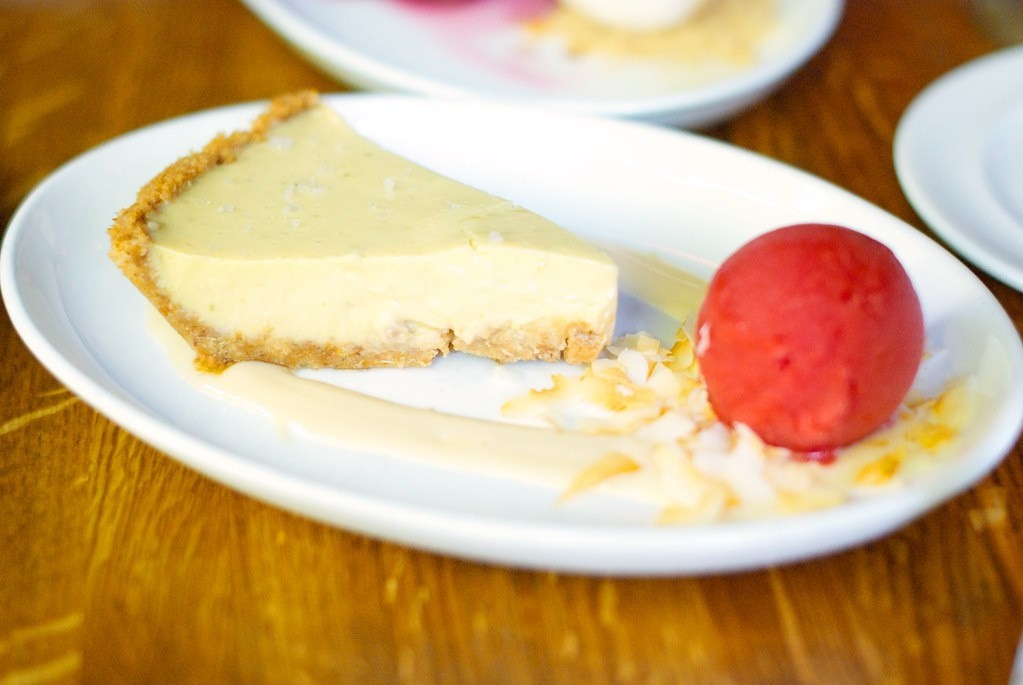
[891,42,1023,295]
[1,93,1022,580]
[247,0,842,123]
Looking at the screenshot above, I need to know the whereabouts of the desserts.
[102,89,617,374]
[691,221,925,460]
